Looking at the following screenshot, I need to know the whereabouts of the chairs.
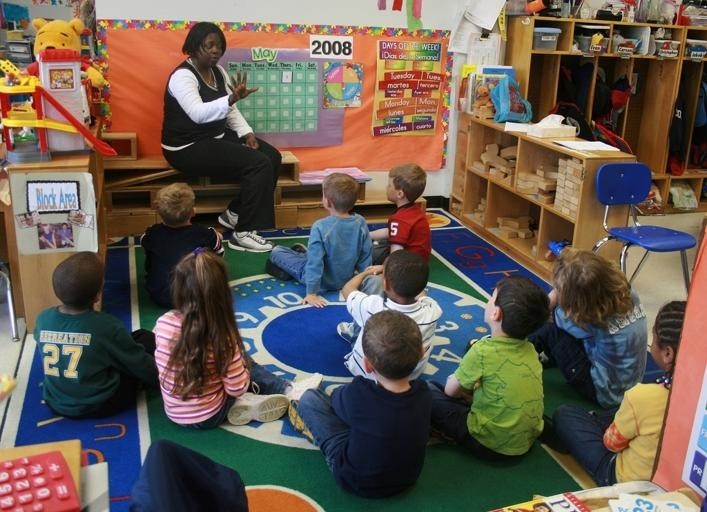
[588,162,698,298]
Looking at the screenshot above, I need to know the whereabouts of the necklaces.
[190,58,214,84]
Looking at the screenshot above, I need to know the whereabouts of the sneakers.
[227,232,272,253]
[287,400,319,446]
[292,243,308,254]
[217,209,257,234]
[227,382,289,426]
[337,322,356,344]
[286,372,324,402]
[266,259,293,281]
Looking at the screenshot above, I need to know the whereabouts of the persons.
[142,184,225,307]
[161,24,282,251]
[37,222,74,249]
[289,310,433,500]
[266,173,373,308]
[33,251,159,419]
[545,300,687,486]
[337,250,443,384]
[428,276,549,459]
[369,163,432,264]
[527,247,647,409]
[152,248,324,429]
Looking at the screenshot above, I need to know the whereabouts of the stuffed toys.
[0,18,119,159]
[474,86,492,108]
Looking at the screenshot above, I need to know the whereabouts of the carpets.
[0,208,668,511]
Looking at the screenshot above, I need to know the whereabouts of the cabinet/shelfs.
[503,13,706,217]
[99,132,427,236]
[448,109,637,286]
[0,119,108,336]
